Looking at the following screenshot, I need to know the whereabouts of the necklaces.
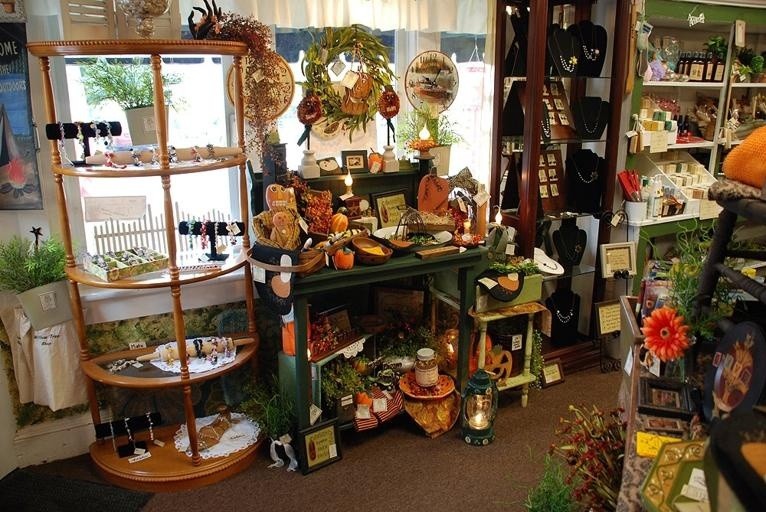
[540,102,551,137]
[570,155,600,184]
[549,293,576,323]
[579,26,600,61]
[552,28,578,73]
[579,101,603,133]
[558,229,582,262]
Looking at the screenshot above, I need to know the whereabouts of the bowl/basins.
[352,237,394,263]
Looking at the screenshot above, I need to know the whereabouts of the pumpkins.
[329,213,349,233]
[336,247,355,269]
[355,389,372,408]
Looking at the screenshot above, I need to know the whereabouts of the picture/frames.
[370,187,412,232]
[636,374,691,419]
[594,296,621,342]
[297,417,342,475]
[341,150,368,173]
[601,241,638,278]
[538,356,564,389]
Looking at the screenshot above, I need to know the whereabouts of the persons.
[186,0,224,39]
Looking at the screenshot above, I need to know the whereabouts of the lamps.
[459,365,499,447]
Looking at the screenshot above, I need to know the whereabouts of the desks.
[217,240,491,466]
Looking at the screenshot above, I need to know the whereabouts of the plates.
[398,372,456,400]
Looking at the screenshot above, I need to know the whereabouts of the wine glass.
[625,201,648,221]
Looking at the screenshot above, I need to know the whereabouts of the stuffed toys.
[251,180,303,251]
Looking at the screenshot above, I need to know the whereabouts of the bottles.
[673,114,689,135]
[414,348,438,388]
[640,173,665,218]
[676,51,725,82]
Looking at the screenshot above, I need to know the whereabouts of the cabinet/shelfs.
[26,38,269,495]
[714,23,766,179]
[429,285,546,409]
[595,16,736,360]
[487,1,633,392]
